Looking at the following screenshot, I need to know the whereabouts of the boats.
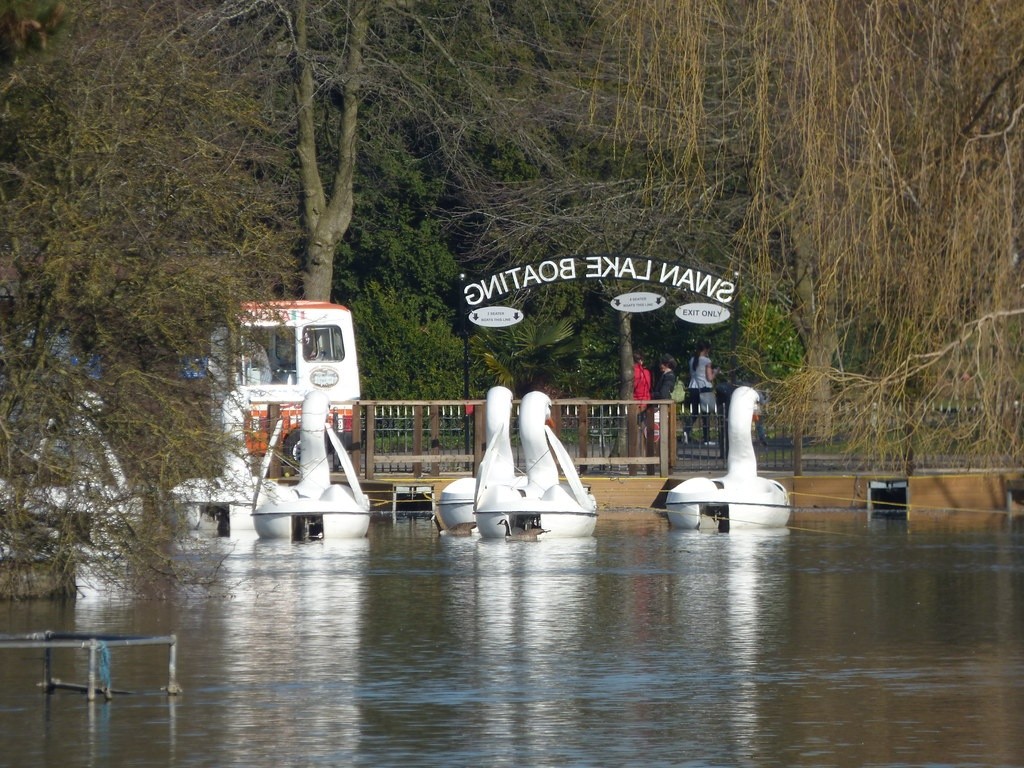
[663,384,792,535]
[433,386,592,532]
[249,390,374,542]
[471,389,598,541]
[0,389,146,562]
[166,384,284,532]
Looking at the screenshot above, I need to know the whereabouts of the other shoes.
[682,432,689,445]
[704,440,718,446]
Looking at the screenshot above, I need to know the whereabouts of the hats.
[661,353,677,368]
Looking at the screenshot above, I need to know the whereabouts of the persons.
[752,380,770,445]
[681,341,720,445]
[633,354,677,470]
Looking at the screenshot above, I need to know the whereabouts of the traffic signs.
[468,306,524,328]
[609,292,667,313]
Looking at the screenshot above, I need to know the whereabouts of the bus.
[24,296,364,469]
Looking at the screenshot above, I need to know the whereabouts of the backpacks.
[665,373,685,402]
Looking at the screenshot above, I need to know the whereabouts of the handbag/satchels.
[699,391,716,414]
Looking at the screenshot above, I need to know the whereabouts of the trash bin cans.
[641,407,660,456]
